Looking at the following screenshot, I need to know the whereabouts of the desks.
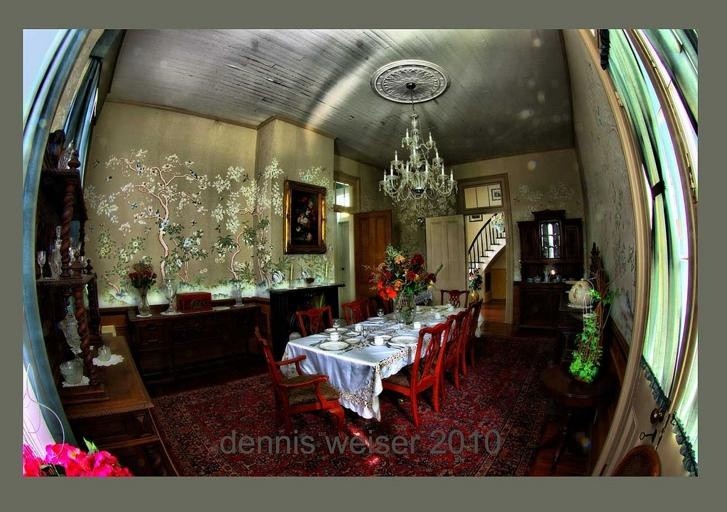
[535,363,616,469]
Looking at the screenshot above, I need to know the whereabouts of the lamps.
[367,59,459,229]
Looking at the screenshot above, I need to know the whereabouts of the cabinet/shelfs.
[34,134,161,466]
[127,303,259,392]
[510,281,567,336]
[516,209,587,281]
[267,283,346,368]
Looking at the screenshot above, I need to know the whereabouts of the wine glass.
[423,300,428,308]
[36,251,46,282]
[53,250,63,275]
[376,309,384,323]
[332,319,340,333]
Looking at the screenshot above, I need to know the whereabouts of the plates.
[318,341,350,351]
[382,334,392,341]
[388,335,419,346]
[345,338,360,344]
[371,342,385,346]
[345,331,360,337]
[324,327,347,334]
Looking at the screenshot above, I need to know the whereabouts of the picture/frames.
[490,188,502,201]
[468,212,484,222]
[281,178,328,255]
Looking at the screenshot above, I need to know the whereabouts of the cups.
[447,306,454,312]
[434,313,441,319]
[373,336,384,345]
[354,324,363,332]
[413,322,421,329]
[328,332,342,342]
[98,346,112,362]
[60,361,83,384]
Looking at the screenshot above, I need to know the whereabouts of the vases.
[134,286,154,318]
[392,287,417,326]
[469,288,480,303]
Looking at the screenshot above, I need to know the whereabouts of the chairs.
[289,304,341,336]
[380,322,451,434]
[457,297,484,378]
[382,297,392,315]
[436,308,470,409]
[341,299,381,328]
[440,289,471,308]
[610,444,665,477]
[252,326,349,443]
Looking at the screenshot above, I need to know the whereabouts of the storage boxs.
[175,292,212,313]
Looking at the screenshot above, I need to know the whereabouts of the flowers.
[23,438,135,477]
[468,273,484,290]
[121,258,161,319]
[359,242,443,326]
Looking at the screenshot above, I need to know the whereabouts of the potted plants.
[560,275,611,383]
